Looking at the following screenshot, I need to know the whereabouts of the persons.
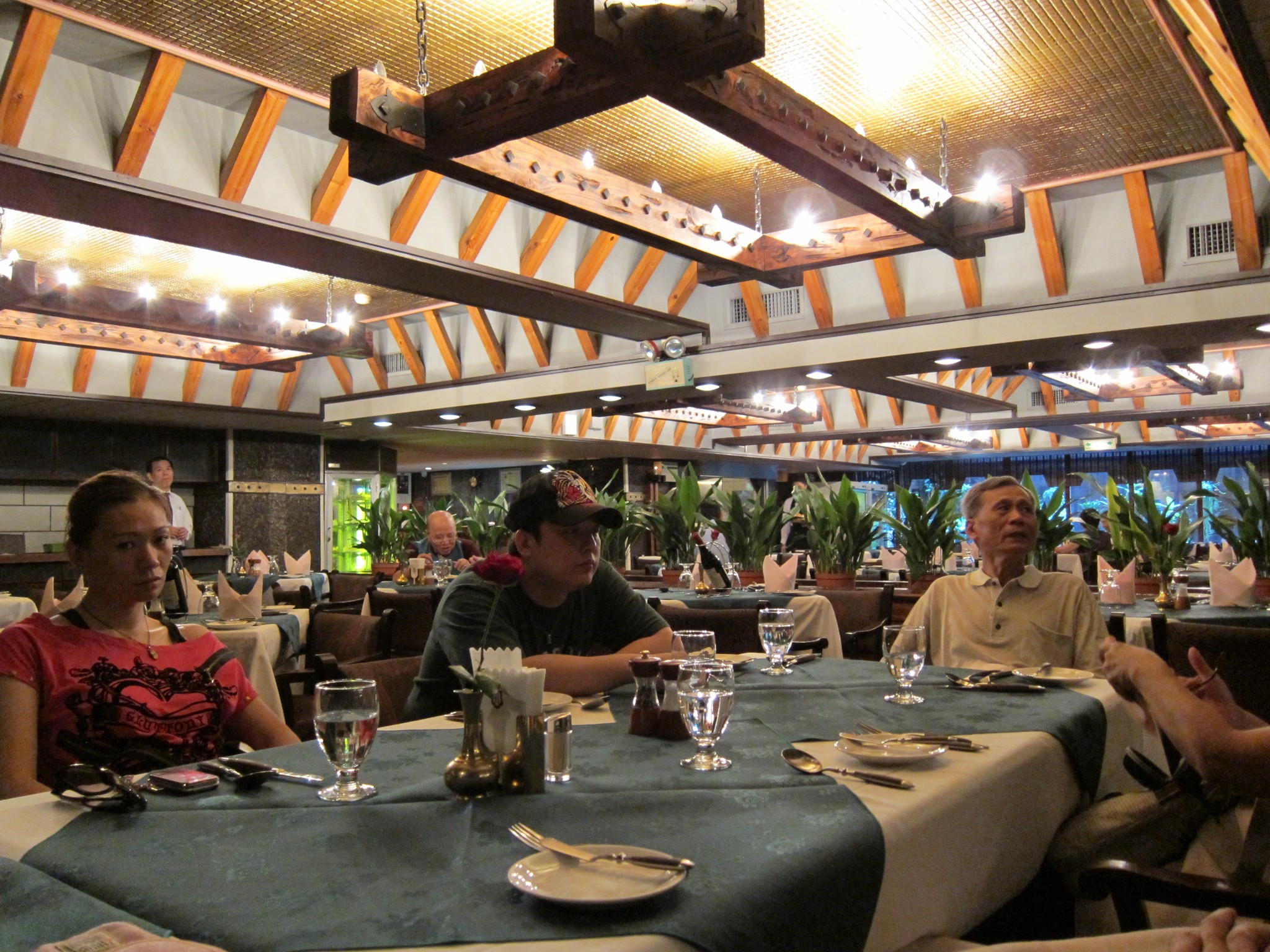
[146,457,193,569]
[879,476,1109,681]
[469,555,486,563]
[393,511,483,584]
[400,470,693,723]
[1080,507,1112,550]
[693,501,732,565]
[958,640,1270,947]
[893,906,1270,952]
[0,470,302,805]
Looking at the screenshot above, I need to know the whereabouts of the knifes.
[540,837,696,873]
[839,732,983,751]
[218,756,324,783]
[936,684,1045,693]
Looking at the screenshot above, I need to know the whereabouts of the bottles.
[628,650,692,741]
[1172,576,1191,610]
[160,559,188,618]
[543,712,573,782]
[691,531,731,593]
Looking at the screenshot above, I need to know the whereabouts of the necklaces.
[81,597,158,660]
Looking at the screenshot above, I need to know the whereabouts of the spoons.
[780,747,916,790]
[197,761,275,788]
[945,671,1000,686]
[571,695,611,710]
[766,653,822,666]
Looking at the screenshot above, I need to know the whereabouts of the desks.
[0,651,1147,951]
[1097,599,1270,628]
[193,572,331,613]
[362,578,449,620]
[635,588,844,659]
[184,610,311,725]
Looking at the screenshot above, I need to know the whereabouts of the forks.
[855,720,990,749]
[507,822,547,851]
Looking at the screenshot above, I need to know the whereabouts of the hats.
[509,468,622,531]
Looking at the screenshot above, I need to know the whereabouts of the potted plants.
[347,483,417,578]
[600,471,1270,605]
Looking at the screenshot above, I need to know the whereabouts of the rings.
[463,565,466,568]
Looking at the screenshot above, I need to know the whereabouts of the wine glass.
[311,678,382,802]
[197,580,220,627]
[671,607,794,772]
[408,556,453,587]
[1098,568,1122,610]
[231,555,280,579]
[881,624,927,705]
[961,548,976,571]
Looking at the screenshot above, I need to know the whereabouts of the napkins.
[1209,539,1234,565]
[691,553,712,589]
[178,568,203,614]
[218,569,263,621]
[1097,554,1137,603]
[283,551,312,576]
[881,545,906,571]
[245,550,270,574]
[40,574,84,618]
[1207,555,1259,608]
[762,555,799,592]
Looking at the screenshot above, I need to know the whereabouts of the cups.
[677,563,742,595]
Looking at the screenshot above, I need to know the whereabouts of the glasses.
[429,533,458,545]
[50,764,148,811]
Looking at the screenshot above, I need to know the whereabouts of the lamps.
[1,0,1270,454]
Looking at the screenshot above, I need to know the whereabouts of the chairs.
[266,581,430,741]
[1108,613,1270,720]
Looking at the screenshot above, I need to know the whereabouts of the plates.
[1011,666,1095,689]
[781,589,817,596]
[541,690,574,712]
[1187,593,1210,602]
[207,620,257,630]
[834,731,949,765]
[687,653,754,668]
[507,844,688,910]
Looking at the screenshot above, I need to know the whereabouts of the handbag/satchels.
[1040,765,1218,896]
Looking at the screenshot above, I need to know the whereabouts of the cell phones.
[148,769,219,793]
